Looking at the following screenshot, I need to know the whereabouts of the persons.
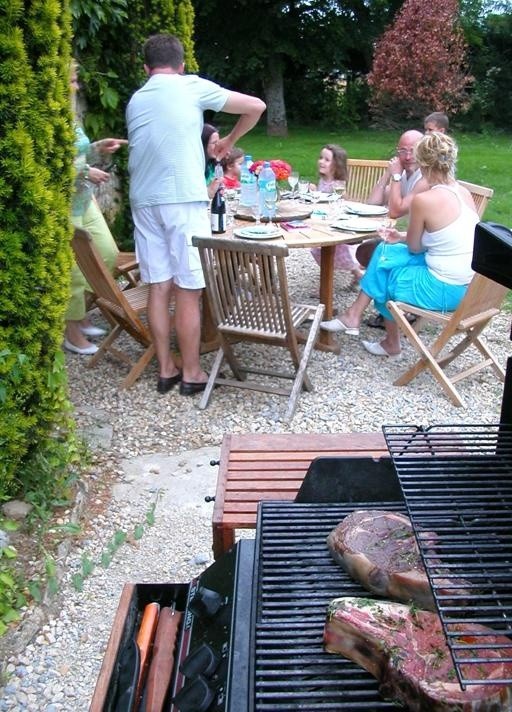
[60,65,129,355]
[201,122,252,302]
[424,111,454,178]
[355,129,426,329]
[221,147,244,190]
[124,34,267,395]
[319,130,475,355]
[308,145,364,290]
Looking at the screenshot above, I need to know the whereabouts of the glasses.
[397,148,414,156]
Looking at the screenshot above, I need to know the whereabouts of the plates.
[233,221,283,239]
[331,215,396,232]
[343,202,390,216]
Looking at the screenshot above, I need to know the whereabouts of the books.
[281,222,310,232]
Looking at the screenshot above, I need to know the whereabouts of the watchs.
[390,173,402,182]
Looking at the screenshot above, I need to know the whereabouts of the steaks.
[324,597,512,712]
[327,508,474,611]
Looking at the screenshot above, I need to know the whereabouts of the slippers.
[320,319,360,337]
[363,339,401,359]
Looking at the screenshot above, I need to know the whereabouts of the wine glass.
[280,170,349,224]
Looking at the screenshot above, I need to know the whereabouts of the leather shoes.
[180,370,226,396]
[81,323,106,339]
[157,367,182,395]
[64,338,100,355]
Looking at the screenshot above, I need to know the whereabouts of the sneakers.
[368,313,416,329]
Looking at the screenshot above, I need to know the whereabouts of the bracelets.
[83,162,89,179]
[376,180,385,189]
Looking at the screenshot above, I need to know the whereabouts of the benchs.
[211,434,465,555]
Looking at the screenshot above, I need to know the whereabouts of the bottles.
[207,153,278,236]
